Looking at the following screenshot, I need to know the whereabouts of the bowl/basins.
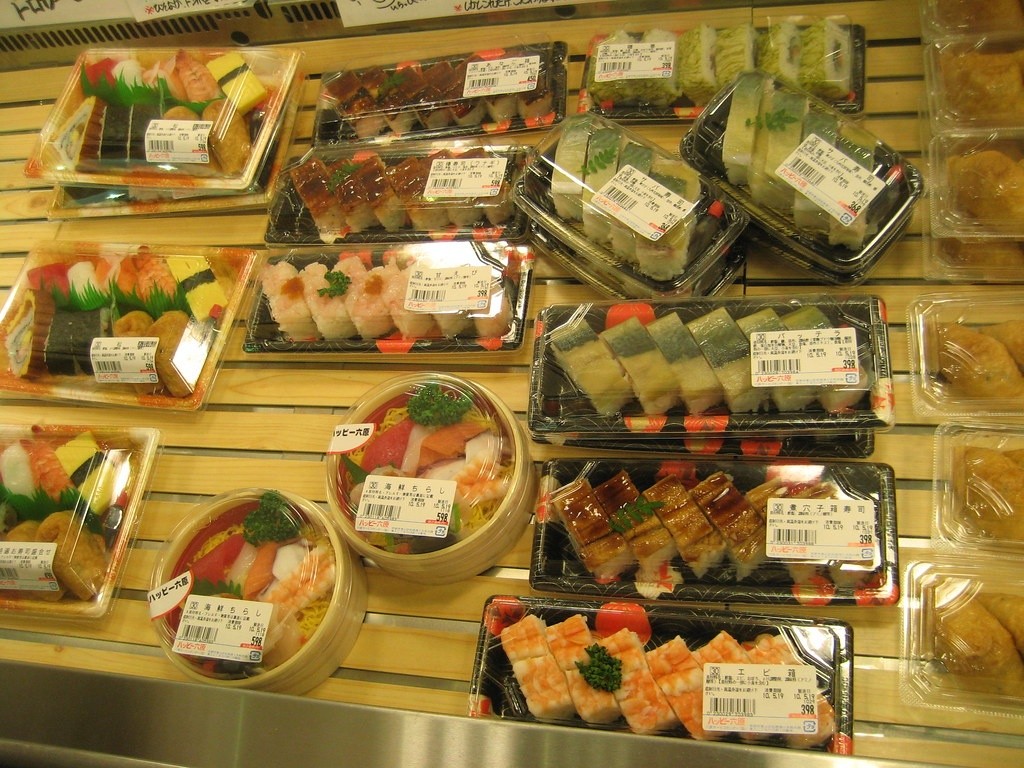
[0,0,1024,768]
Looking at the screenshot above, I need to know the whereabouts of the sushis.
[0,0,1024,747]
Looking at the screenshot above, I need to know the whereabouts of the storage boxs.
[1,0,1024,756]
